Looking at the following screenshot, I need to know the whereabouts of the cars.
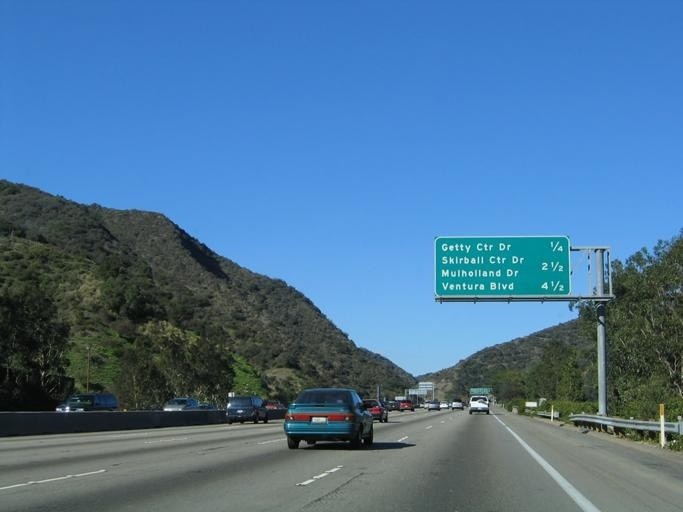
[361,399,388,422]
[380,400,413,412]
[470,396,489,414]
[164,398,197,410]
[419,398,463,411]
[284,388,373,449]
[226,397,268,423]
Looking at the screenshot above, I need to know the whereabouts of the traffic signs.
[434,236,570,296]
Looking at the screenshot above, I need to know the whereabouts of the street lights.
[86,346,90,392]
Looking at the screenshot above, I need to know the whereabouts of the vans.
[55,391,117,412]
